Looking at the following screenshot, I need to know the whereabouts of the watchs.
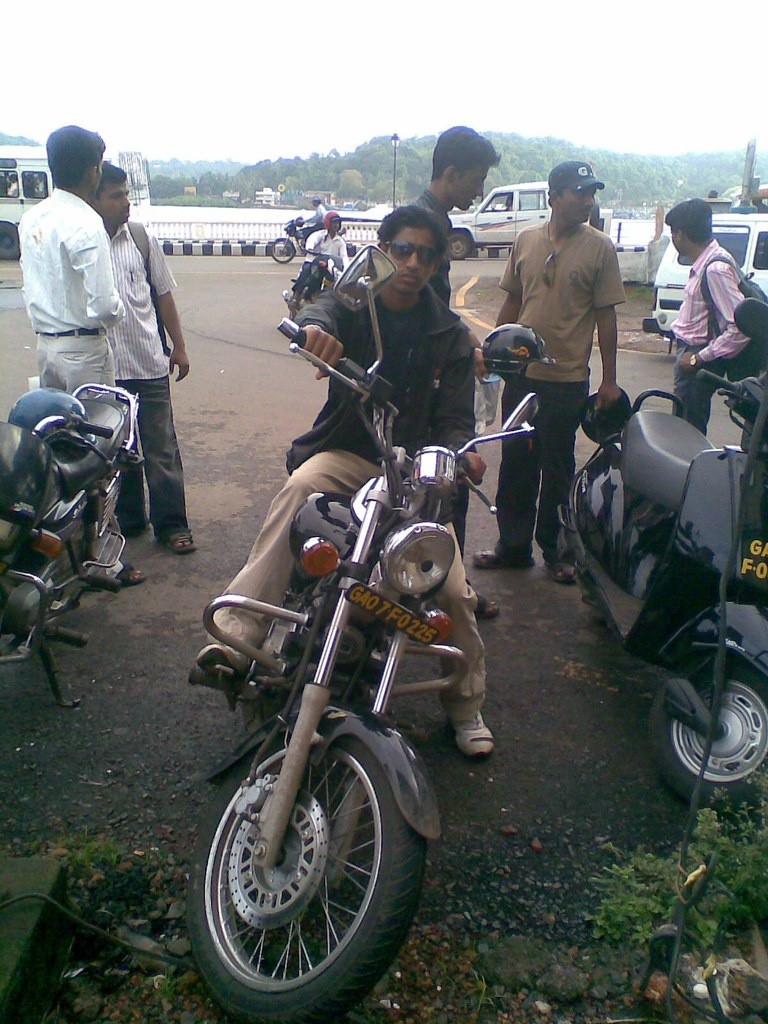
[689,354,699,370]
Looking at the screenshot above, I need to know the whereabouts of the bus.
[0,145,120,261]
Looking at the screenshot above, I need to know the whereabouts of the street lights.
[391,133,400,211]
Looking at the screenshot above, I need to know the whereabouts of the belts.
[44,328,107,336]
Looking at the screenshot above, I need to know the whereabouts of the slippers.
[552,562,577,583]
[474,550,535,568]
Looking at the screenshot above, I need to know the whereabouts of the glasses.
[542,253,555,288]
[385,239,435,262]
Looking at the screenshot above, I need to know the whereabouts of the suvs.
[448,181,600,261]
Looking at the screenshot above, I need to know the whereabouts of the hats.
[548,160,604,195]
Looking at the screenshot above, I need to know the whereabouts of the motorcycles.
[557,298,768,816]
[1,382,145,709]
[189,245,540,1024]
[270,217,347,264]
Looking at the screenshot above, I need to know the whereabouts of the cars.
[642,213,768,382]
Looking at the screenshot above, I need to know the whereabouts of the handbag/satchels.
[701,256,768,381]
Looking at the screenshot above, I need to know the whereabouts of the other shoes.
[293,290,299,299]
[456,723,494,758]
[197,643,249,677]
[115,564,146,586]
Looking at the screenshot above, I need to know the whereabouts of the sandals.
[165,531,197,555]
[474,595,499,619]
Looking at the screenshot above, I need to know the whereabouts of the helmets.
[581,386,631,444]
[481,322,556,372]
[313,197,321,204]
[325,212,342,231]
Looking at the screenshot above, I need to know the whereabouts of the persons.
[287,213,351,312]
[297,196,327,250]
[90,161,198,554]
[615,207,636,219]
[197,205,500,758]
[18,125,147,584]
[7,174,45,197]
[665,198,751,435]
[473,162,622,584]
[396,127,502,310]
